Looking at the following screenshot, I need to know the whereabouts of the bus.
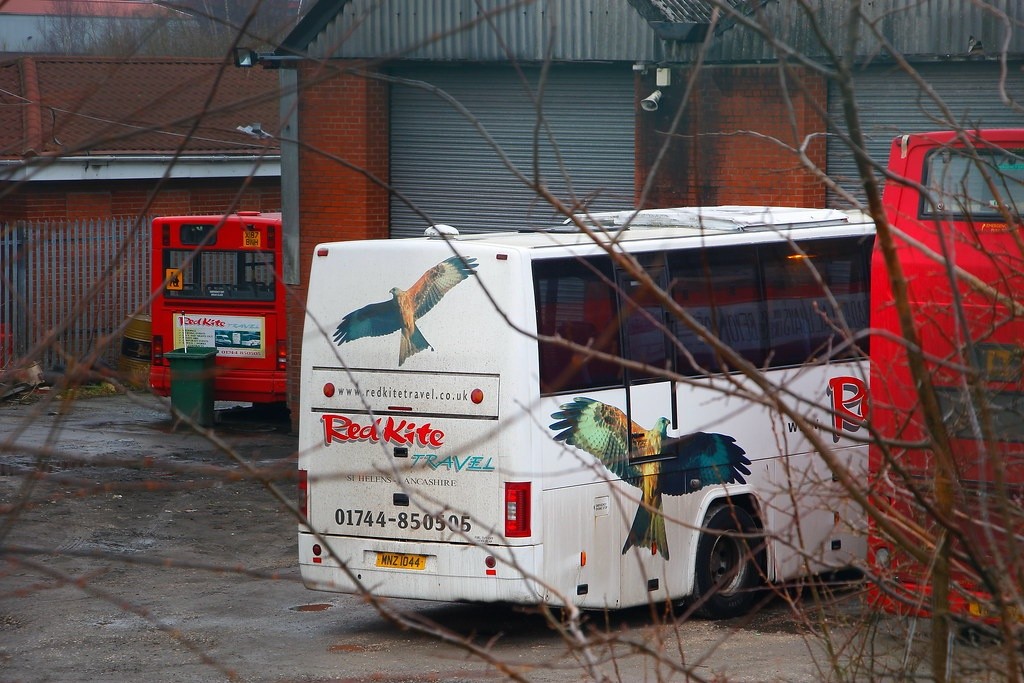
[149,210,287,403]
[295,203,878,621]
[866,126,1024,632]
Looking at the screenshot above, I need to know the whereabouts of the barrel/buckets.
[119,313,152,393]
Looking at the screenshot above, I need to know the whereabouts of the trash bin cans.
[162,347,219,426]
